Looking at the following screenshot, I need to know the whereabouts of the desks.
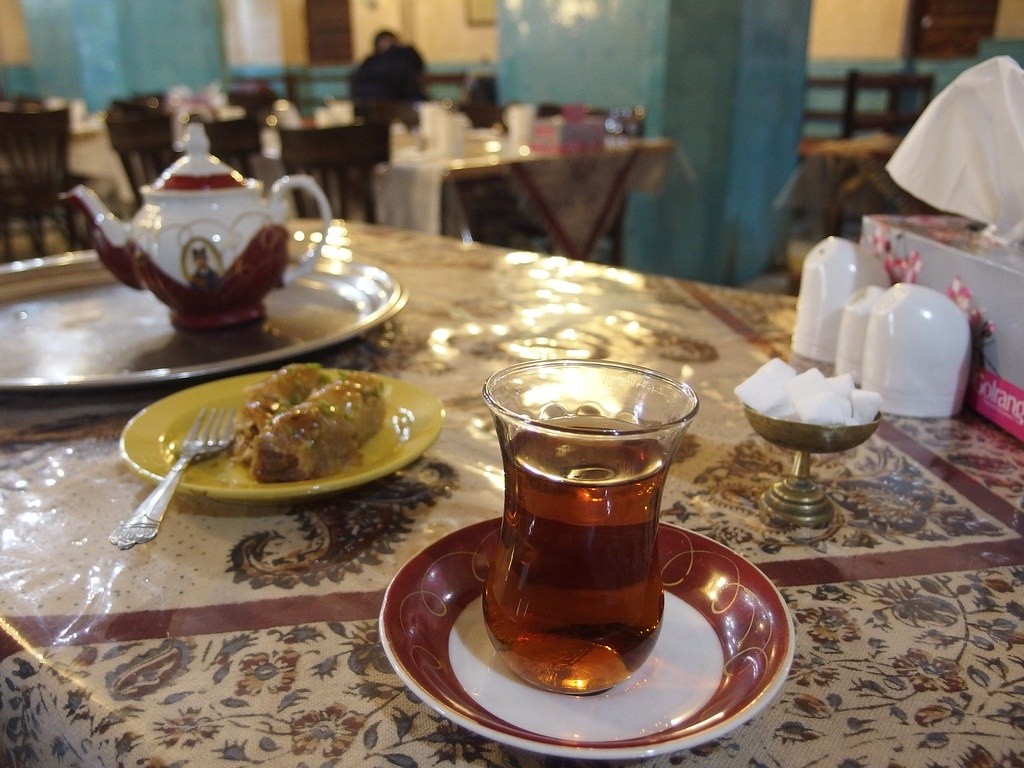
[369,135,677,269]
[788,137,930,241]
[0,219,1024,768]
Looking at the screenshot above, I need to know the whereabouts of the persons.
[348,32,431,126]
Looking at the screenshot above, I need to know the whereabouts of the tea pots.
[57,122,332,331]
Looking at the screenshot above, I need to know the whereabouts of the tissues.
[530,105,605,154]
[857,53,1024,444]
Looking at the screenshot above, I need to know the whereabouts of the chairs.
[0,78,507,268]
[797,69,936,140]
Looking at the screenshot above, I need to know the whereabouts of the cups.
[418,102,464,159]
[506,103,536,151]
[790,234,972,419]
[481,357,699,697]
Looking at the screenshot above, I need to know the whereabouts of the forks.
[106,406,237,551]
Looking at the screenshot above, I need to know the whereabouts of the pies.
[229,361,386,481]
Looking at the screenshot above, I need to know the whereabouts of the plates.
[378,515,796,762]
[116,369,447,503]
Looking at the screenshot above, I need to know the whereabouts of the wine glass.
[743,403,885,528]
